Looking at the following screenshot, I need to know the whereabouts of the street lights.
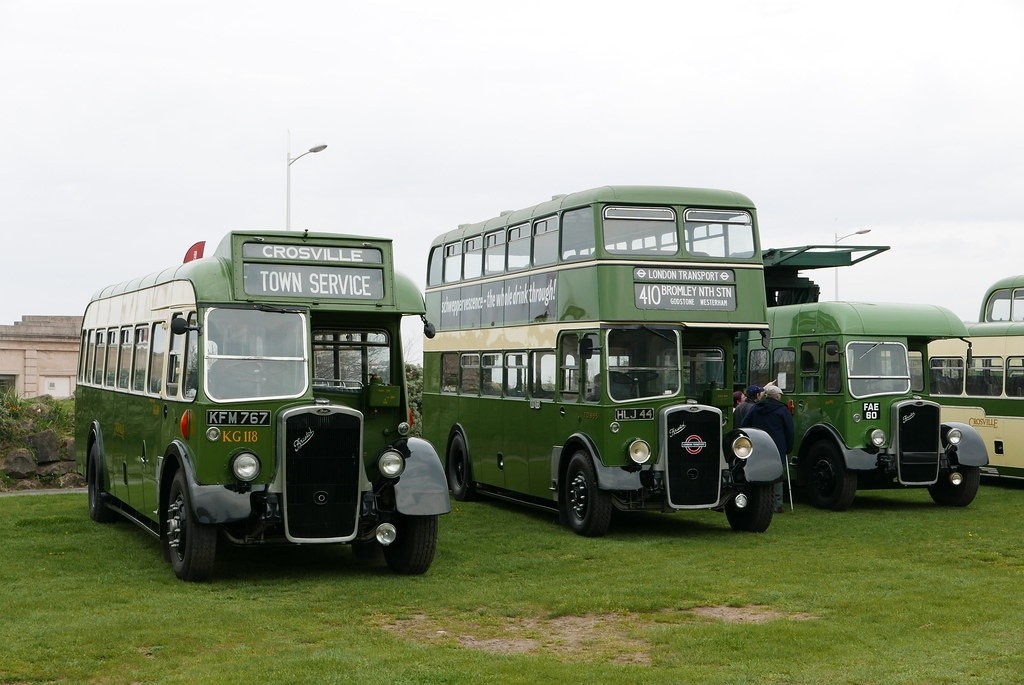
[835,230,871,301]
[287,145,327,231]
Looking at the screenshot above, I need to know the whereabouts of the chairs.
[940,375,1024,397]
[596,371,637,402]
[825,361,841,391]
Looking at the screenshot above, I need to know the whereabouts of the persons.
[731,384,795,512]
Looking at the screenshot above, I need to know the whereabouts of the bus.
[422,186,783,538]
[979,275,1024,322]
[665,246,989,510]
[881,321,1024,479]
[74,228,451,581]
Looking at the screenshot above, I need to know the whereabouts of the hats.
[763,385,784,395]
[746,385,765,395]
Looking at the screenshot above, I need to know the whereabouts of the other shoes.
[774,506,784,513]
